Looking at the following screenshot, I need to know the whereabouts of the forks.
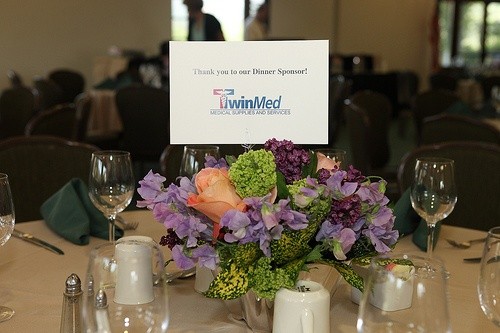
[110,212,140,232]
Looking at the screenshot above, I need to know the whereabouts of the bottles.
[58,273,111,333]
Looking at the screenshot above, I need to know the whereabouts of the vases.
[221,257,350,330]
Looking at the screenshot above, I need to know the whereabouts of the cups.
[81,236,170,333]
[354,252,451,332]
[180,143,223,183]
[270,278,330,333]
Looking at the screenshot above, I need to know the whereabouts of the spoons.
[445,237,487,249]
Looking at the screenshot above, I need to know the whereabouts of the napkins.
[380,184,441,253]
[37,180,123,247]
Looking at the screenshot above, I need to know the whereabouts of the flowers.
[138,142,407,298]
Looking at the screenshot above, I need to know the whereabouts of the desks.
[83,87,121,136]
[0,208,499,333]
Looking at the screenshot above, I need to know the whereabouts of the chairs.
[0,58,499,234]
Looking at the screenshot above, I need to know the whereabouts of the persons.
[183,0,226,41]
[148,40,168,86]
[117,56,147,85]
[244,4,267,41]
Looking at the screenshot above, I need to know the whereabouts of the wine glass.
[87,151,135,249]
[0,173,15,322]
[405,156,458,276]
[478,226,500,329]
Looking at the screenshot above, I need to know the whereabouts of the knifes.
[463,256,500,264]
[6,229,65,256]
